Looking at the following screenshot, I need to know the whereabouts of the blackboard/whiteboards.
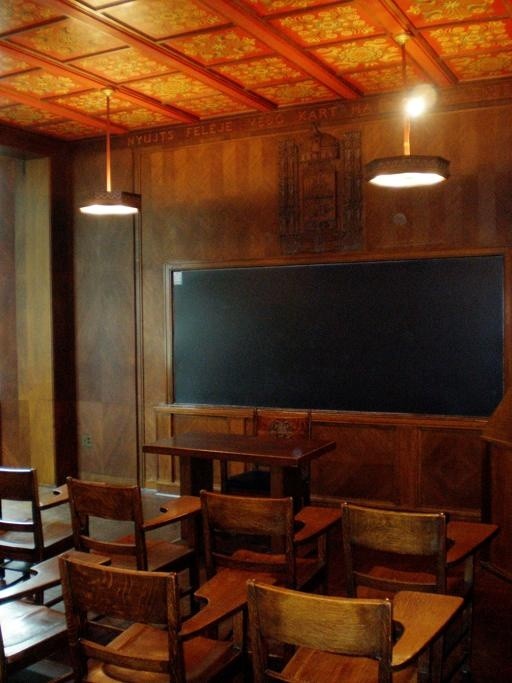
[163,246,511,429]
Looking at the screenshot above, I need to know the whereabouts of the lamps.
[360,28,452,192]
[76,86,142,217]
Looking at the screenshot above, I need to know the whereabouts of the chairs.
[57,552,274,683]
[341,502,499,680]
[246,580,463,682]
[65,476,202,624]
[219,411,315,530]
[198,489,340,598]
[2,467,70,604]
[1,550,110,683]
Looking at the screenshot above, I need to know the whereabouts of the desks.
[141,430,336,566]
